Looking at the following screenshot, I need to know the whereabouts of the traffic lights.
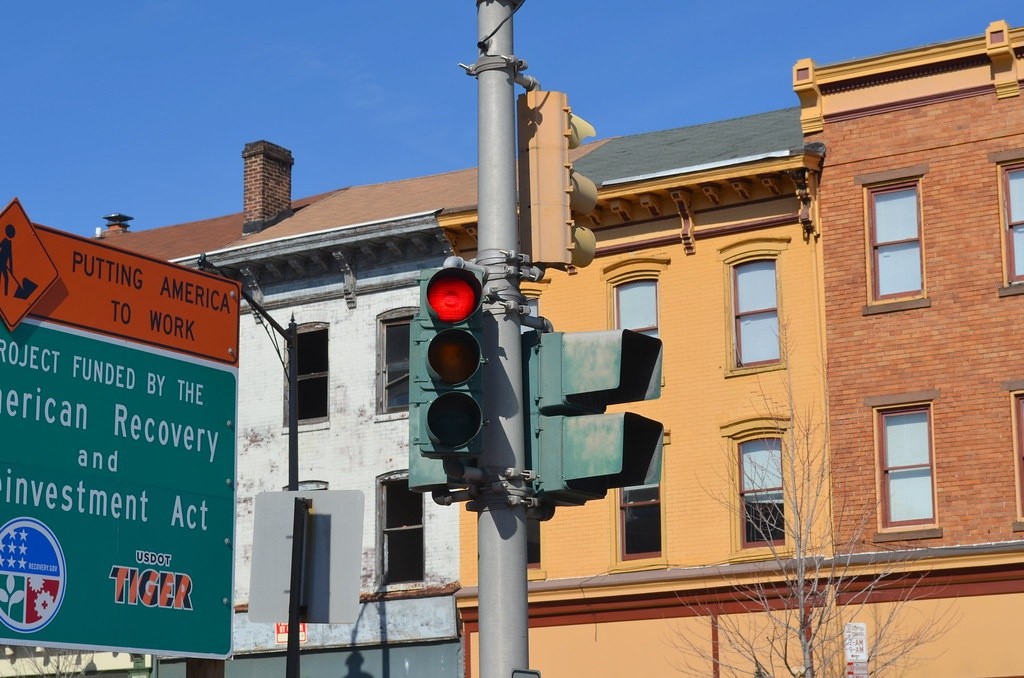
[537,329,664,512]
[515,90,601,269]
[417,265,487,459]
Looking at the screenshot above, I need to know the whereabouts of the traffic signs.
[0,197,242,662]
